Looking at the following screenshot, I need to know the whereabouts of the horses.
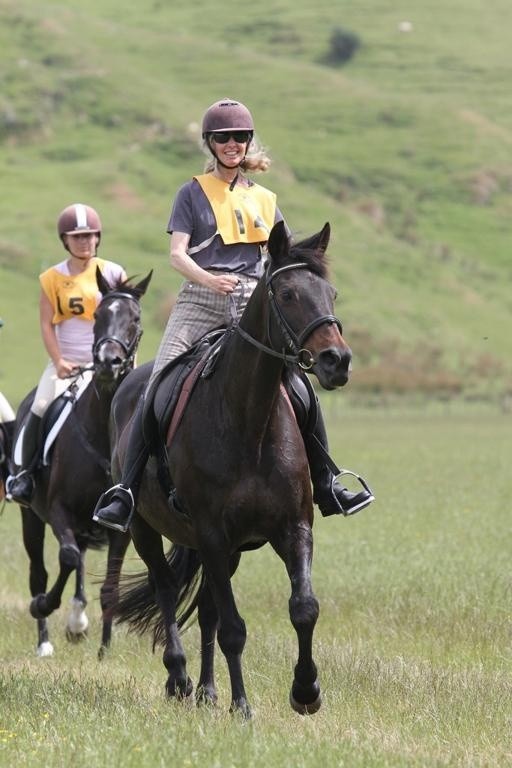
[0,263,155,662]
[108,219,355,737]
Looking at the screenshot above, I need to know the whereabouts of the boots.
[97,400,148,524]
[302,400,370,517]
[12,409,41,503]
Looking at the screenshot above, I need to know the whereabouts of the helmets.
[58,203,101,235]
[202,100,255,139]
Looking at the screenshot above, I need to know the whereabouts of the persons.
[5,204,130,504]
[98,100,369,523]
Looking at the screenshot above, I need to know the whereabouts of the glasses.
[214,133,248,143]
